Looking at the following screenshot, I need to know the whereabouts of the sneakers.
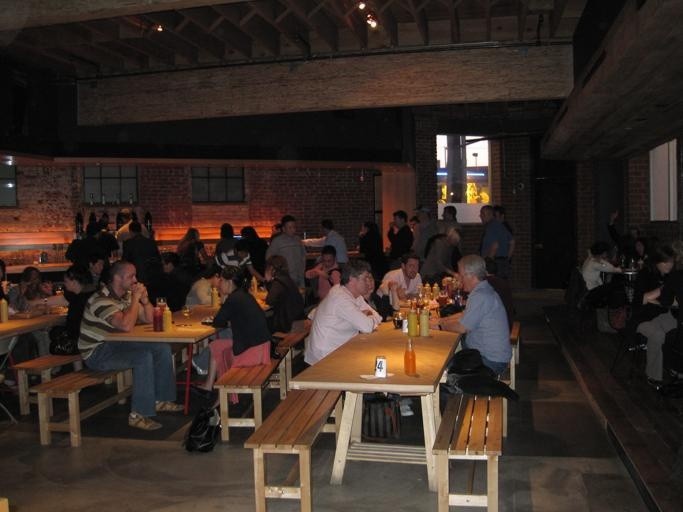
[128,414,162,430]
[156,401,184,412]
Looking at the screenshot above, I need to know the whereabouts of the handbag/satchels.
[457,376,519,402]
[271,335,291,359]
[49,326,79,356]
[577,292,607,311]
[185,408,220,452]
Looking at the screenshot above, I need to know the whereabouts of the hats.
[412,205,432,214]
[451,348,484,373]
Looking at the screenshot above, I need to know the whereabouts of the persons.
[302,219,399,369]
[480,205,514,278]
[191,266,272,409]
[265,256,304,340]
[265,215,307,291]
[565,210,682,389]
[377,205,464,306]
[6,209,166,374]
[78,261,185,431]
[162,223,267,374]
[426,255,511,412]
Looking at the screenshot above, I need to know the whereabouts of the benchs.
[272,319,312,392]
[12,354,84,416]
[243,390,343,512]
[500,321,521,390]
[28,368,133,447]
[213,348,289,442]
[434,396,508,512]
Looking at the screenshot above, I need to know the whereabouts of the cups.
[393,318,403,330]
[446,277,453,296]
[438,296,447,307]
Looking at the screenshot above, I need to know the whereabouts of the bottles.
[0,298,8,323]
[89,191,134,206]
[38,251,48,264]
[250,275,257,293]
[619,254,644,272]
[152,297,173,333]
[415,283,441,309]
[406,307,417,336]
[57,287,63,297]
[419,309,429,337]
[42,297,49,314]
[403,338,416,377]
[210,287,219,308]
[51,243,64,263]
[303,230,311,239]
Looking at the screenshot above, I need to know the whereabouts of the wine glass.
[181,304,193,326]
